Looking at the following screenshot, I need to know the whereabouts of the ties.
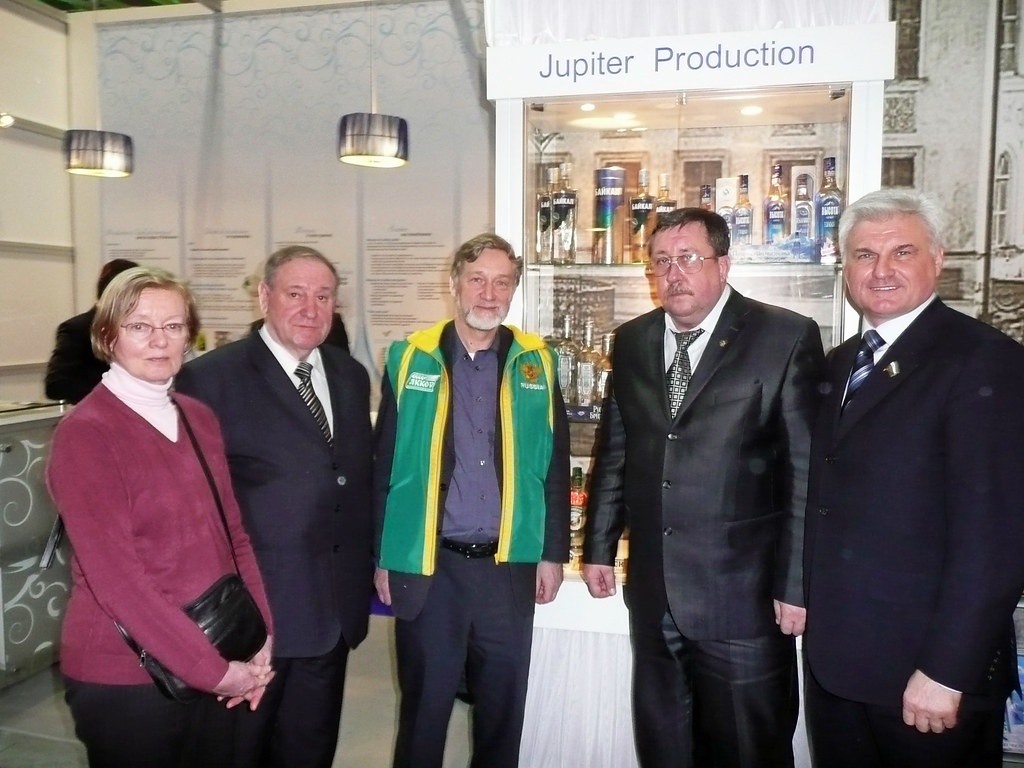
[841,330,886,416]
[666,328,707,419]
[293,361,334,450]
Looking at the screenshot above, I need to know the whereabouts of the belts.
[437,536,500,558]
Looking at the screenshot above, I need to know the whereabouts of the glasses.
[648,255,719,277]
[118,322,190,338]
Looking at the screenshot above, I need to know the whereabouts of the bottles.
[562,467,588,548]
[630,169,676,263]
[795,174,809,200]
[538,163,577,266]
[700,184,711,211]
[553,315,614,407]
[814,157,843,265]
[734,174,752,247]
[762,165,787,244]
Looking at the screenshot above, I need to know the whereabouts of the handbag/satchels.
[136,573,268,706]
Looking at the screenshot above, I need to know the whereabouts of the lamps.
[340,0,409,168]
[61,0,134,177]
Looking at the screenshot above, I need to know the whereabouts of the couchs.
[0,414,90,768]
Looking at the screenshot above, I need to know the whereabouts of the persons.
[372,233,570,768]
[46,267,275,768]
[172,247,377,768]
[802,188,1024,768]
[579,207,824,768]
[42,260,144,407]
